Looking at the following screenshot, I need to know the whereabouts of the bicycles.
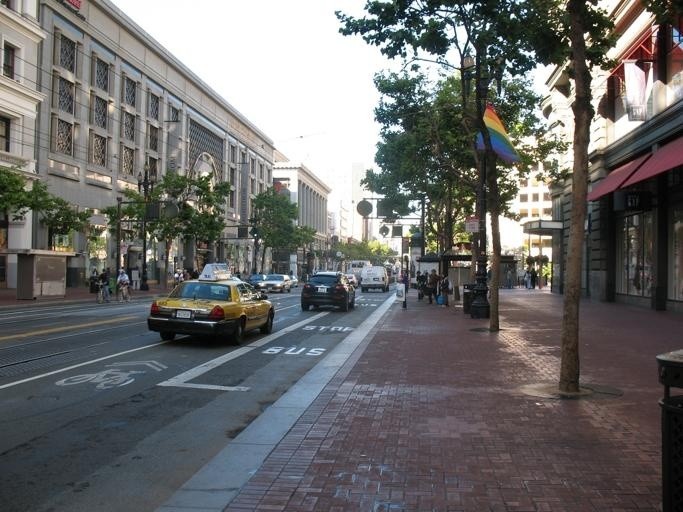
[94,282,112,304]
[116,283,132,304]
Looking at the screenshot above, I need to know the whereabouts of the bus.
[349,260,371,283]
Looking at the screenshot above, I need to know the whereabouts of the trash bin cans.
[463,284,477,314]
[656,349,682,512]
[89,276,100,294]
[536,276,539,286]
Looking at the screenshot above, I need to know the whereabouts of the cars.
[256,274,290,294]
[244,274,266,290]
[289,276,298,287]
[344,274,358,289]
[300,271,355,312]
[147,263,274,345]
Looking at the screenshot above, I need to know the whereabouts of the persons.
[530,268,537,289]
[96,269,111,303]
[524,268,531,288]
[507,268,513,290]
[173,267,201,287]
[487,267,491,279]
[236,269,240,279]
[440,270,449,307]
[404,272,409,294]
[116,269,131,303]
[425,268,443,304]
[92,267,99,276]
[415,270,423,302]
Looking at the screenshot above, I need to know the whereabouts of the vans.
[360,266,388,293]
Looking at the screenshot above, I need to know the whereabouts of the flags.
[473,104,521,169]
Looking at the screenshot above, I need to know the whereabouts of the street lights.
[460,44,504,321]
[136,160,155,291]
[248,212,260,275]
